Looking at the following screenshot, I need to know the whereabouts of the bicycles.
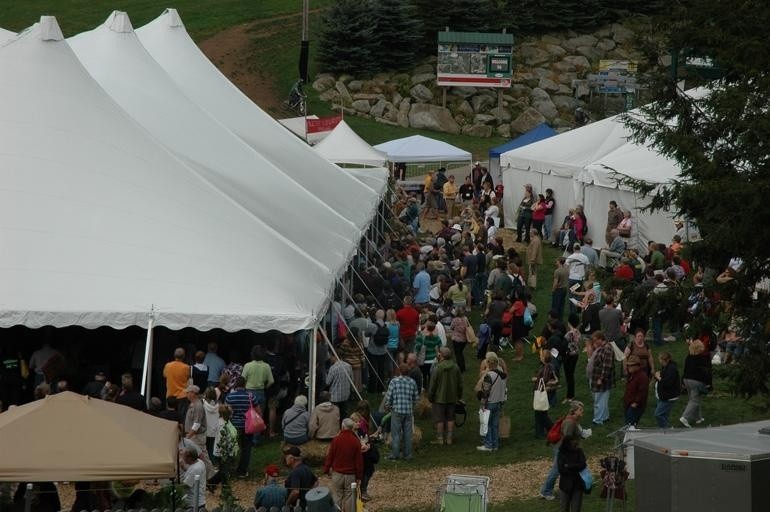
[279,93,307,117]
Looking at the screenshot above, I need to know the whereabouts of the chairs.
[609,251,625,269]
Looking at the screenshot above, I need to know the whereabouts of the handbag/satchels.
[712,350,724,365]
[356,484,363,512]
[245,407,266,434]
[579,467,592,490]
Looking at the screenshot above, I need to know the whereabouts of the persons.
[288,78,305,105]
[438,52,486,74]
[0,152,769,511]
[574,104,596,126]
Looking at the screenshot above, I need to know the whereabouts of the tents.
[1,0,472,422]
[499,77,769,296]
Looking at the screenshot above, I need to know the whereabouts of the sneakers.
[680,417,692,428]
[664,336,676,342]
[696,418,704,424]
[362,434,555,501]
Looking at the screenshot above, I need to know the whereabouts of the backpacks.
[374,323,389,347]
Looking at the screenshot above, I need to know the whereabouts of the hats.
[264,465,279,477]
[183,385,200,394]
[283,448,300,457]
